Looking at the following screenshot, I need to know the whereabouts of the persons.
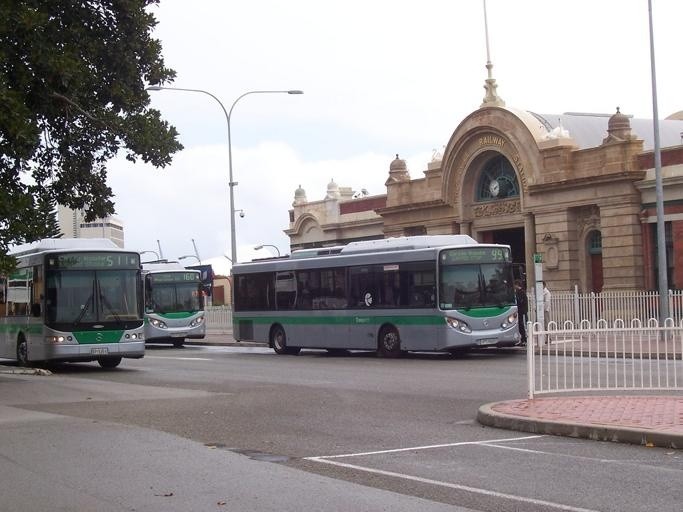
[543,280,552,344]
[514,280,528,346]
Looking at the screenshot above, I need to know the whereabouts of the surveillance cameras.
[354,192,360,198]
[240,213,245,217]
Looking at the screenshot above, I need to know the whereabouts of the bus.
[231,234,522,358]
[0,238,207,365]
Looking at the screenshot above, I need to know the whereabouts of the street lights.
[254,244,280,256]
[145,86,304,264]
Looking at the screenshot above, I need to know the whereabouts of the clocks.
[489,179,499,197]
